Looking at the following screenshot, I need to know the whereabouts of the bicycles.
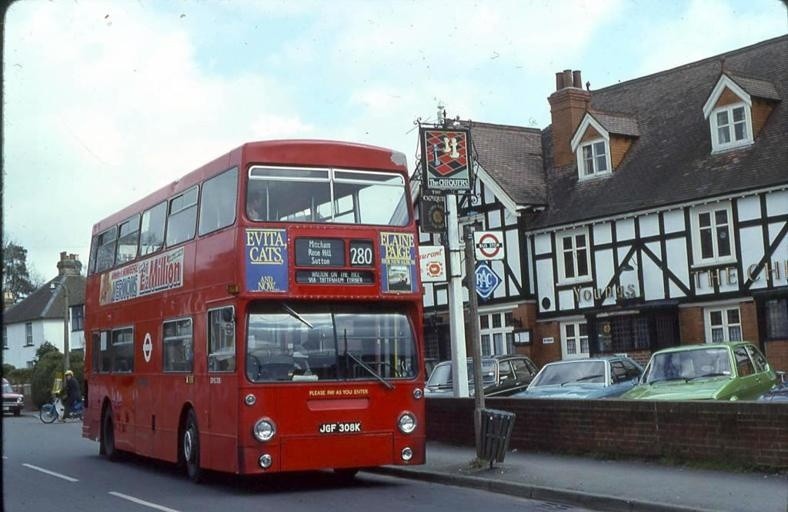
[764,370,788,402]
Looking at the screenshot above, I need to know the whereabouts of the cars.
[1,377,25,417]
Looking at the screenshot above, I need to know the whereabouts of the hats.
[64,370,74,378]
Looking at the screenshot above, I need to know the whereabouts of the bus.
[82,138,426,484]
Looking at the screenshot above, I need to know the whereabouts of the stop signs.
[473,231,503,260]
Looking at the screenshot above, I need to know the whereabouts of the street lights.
[50,280,70,386]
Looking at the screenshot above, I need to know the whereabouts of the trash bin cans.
[476,408,516,469]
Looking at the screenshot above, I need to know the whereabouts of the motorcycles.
[39,390,83,423]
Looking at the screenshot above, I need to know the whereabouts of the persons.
[245,191,263,222]
[55,370,82,424]
